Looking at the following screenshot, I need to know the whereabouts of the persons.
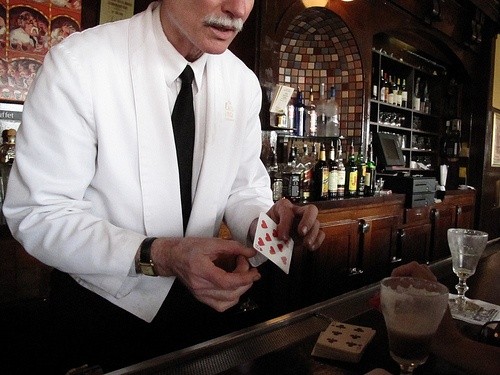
[1,0,324,374]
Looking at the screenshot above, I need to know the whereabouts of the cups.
[0,177,8,204]
[376,180,384,192]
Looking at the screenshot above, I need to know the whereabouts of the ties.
[172,65,196,237]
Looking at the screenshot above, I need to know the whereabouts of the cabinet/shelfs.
[265,190,477,313]
[370,31,459,180]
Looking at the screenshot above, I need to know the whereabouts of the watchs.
[137,237,158,277]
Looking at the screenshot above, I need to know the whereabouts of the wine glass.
[381,277,449,375]
[447,228,489,314]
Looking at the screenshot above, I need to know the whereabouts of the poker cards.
[253,211,295,274]
[311,320,376,363]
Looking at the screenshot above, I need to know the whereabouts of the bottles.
[294,90,306,137]
[372,68,385,101]
[326,86,340,136]
[366,145,376,197]
[413,136,431,151]
[282,144,312,204]
[415,78,431,113]
[317,82,327,136]
[393,77,407,108]
[268,147,282,202]
[379,132,389,135]
[416,156,428,165]
[305,85,318,137]
[390,132,405,148]
[369,132,373,144]
[440,120,461,157]
[0,129,16,173]
[310,142,329,201]
[384,73,394,104]
[328,140,346,200]
[346,145,366,198]
